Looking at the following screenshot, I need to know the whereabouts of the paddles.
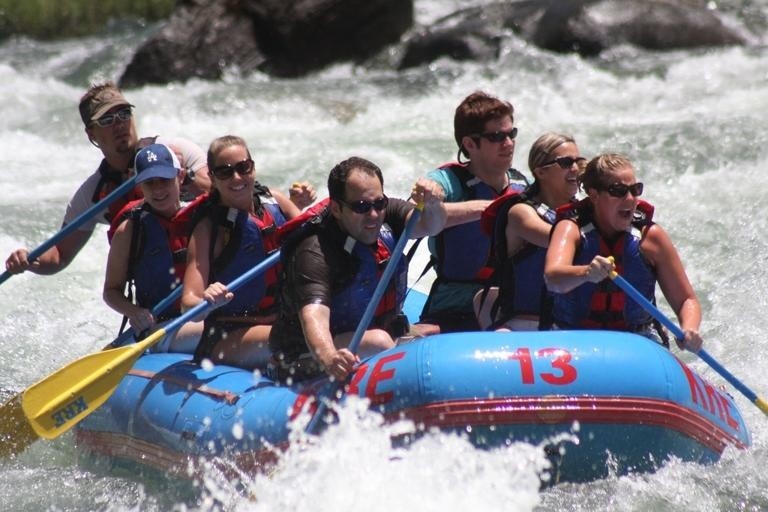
[0,284,184,462]
[21,250,281,439]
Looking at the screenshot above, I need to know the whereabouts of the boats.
[70,286,750,487]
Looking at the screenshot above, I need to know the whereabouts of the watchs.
[183,167,195,185]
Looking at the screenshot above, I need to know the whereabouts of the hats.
[83,84,135,127]
[134,144,181,184]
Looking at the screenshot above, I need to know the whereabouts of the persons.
[267,156,445,387]
[103,145,317,353]
[503,131,587,341]
[542,152,703,353]
[181,134,302,368]
[407,90,536,332]
[6,80,213,277]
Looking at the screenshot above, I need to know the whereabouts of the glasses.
[207,159,255,179]
[89,106,132,128]
[532,157,586,170]
[594,184,643,197]
[457,127,518,165]
[334,194,390,213]
